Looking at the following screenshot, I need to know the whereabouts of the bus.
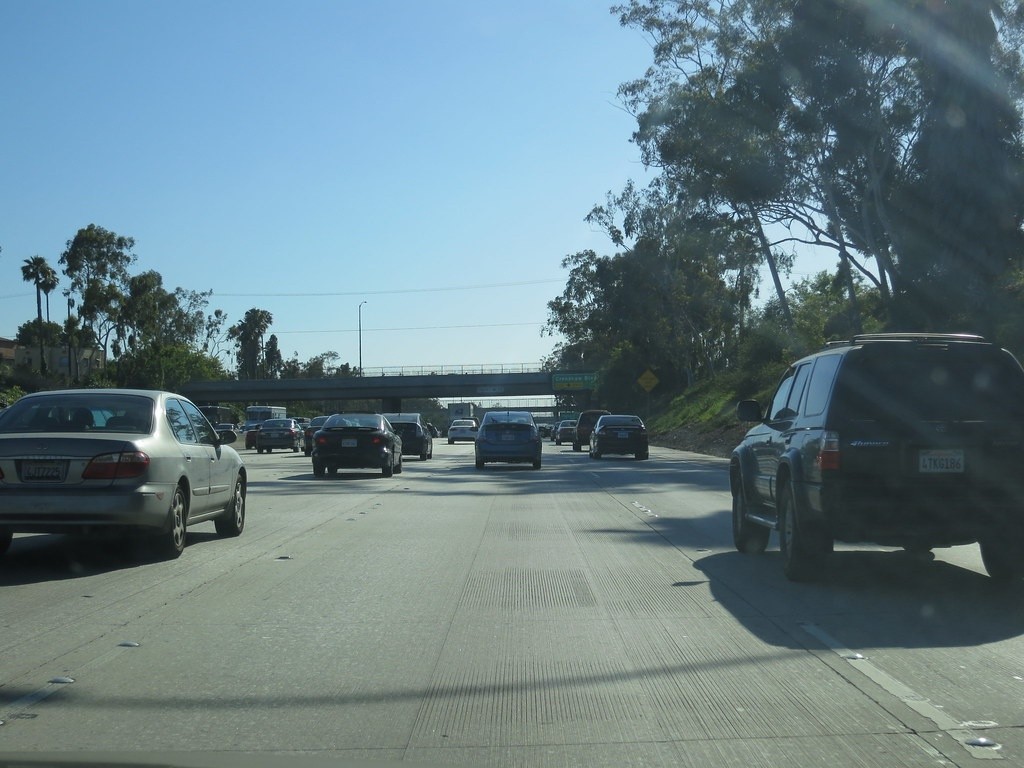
[240,406,287,432]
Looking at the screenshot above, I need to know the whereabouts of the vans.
[570,410,614,452]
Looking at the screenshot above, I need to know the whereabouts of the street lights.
[359,301,367,376]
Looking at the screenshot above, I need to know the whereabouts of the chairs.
[70,407,94,430]
[106,415,146,430]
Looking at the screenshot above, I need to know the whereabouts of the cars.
[383,412,434,461]
[555,420,579,445]
[0,388,248,561]
[311,410,404,478]
[537,422,561,441]
[245,424,263,450]
[255,417,306,455]
[290,417,310,431]
[474,411,543,470]
[448,420,481,444]
[589,415,649,460]
[304,416,332,457]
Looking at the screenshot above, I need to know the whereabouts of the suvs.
[729,333,1024,586]
